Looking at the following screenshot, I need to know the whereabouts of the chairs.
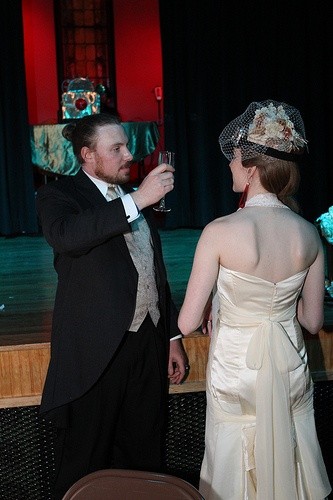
[61,467,206,500]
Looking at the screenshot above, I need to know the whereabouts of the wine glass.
[152,151,175,213]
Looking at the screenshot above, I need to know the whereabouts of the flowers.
[245,102,309,154]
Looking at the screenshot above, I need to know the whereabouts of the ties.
[107,187,118,200]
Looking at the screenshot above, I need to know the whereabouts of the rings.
[185,365,189,369]
[207,320,211,322]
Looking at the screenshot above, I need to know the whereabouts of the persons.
[37,115,190,500]
[177,99,331,500]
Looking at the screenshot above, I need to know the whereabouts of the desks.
[29,120,162,233]
